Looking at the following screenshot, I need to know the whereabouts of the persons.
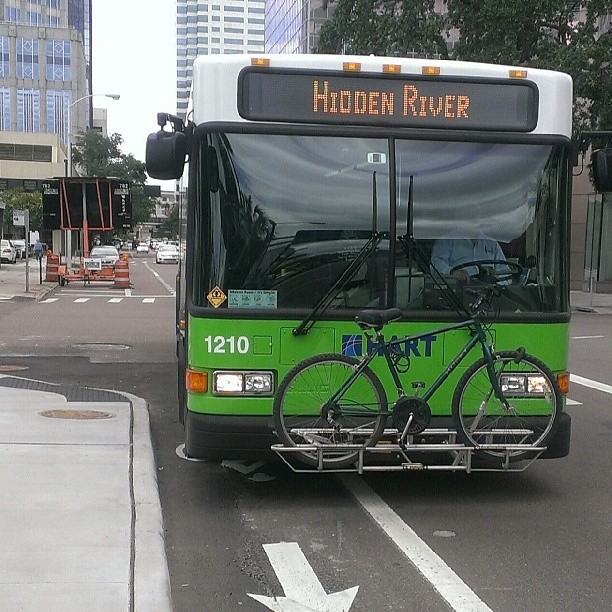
[430,199,513,287]
[35,239,43,261]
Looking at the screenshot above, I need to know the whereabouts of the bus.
[145,52,611,476]
[155,244,181,264]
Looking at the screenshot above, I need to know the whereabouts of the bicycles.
[272,283,564,471]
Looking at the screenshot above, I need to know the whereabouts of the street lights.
[66,93,121,274]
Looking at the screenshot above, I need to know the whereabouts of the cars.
[136,242,149,254]
[0,238,18,263]
[9,239,26,260]
[89,245,120,267]
